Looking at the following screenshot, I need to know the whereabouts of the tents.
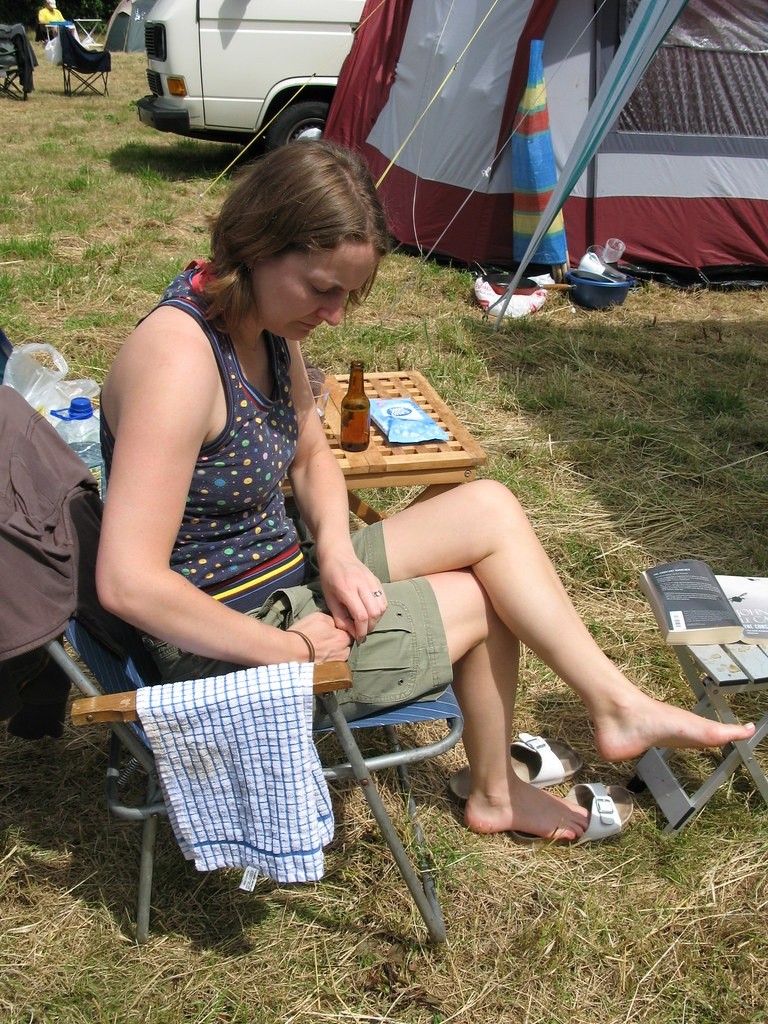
[103,0,156,51]
[319,0,768,277]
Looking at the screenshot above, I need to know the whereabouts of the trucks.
[135,0,369,162]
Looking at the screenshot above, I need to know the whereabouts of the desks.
[74,19,105,47]
[44,25,75,62]
[282,369,488,525]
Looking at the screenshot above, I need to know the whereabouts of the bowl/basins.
[563,272,637,310]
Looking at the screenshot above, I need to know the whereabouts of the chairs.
[36,15,53,45]
[0,385,465,948]
[59,25,111,97]
[0,23,27,101]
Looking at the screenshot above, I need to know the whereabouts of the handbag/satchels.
[2,341,102,431]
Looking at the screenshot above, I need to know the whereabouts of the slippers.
[507,783,635,847]
[448,730,585,804]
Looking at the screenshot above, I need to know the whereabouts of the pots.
[472,260,538,295]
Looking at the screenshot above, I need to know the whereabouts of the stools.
[626,641,768,840]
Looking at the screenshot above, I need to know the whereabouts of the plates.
[572,269,615,284]
[589,255,627,283]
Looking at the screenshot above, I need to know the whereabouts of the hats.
[46,0,57,9]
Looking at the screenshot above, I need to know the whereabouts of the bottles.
[340,362,370,452]
[50,397,107,504]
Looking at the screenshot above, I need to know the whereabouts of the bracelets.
[286,630,315,663]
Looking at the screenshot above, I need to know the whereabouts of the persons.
[96,141,758,845]
[38,0,75,38]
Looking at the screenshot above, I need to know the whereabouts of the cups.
[310,381,330,423]
[604,238,625,262]
[587,245,607,264]
[577,253,604,277]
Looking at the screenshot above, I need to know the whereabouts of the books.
[638,559,768,646]
